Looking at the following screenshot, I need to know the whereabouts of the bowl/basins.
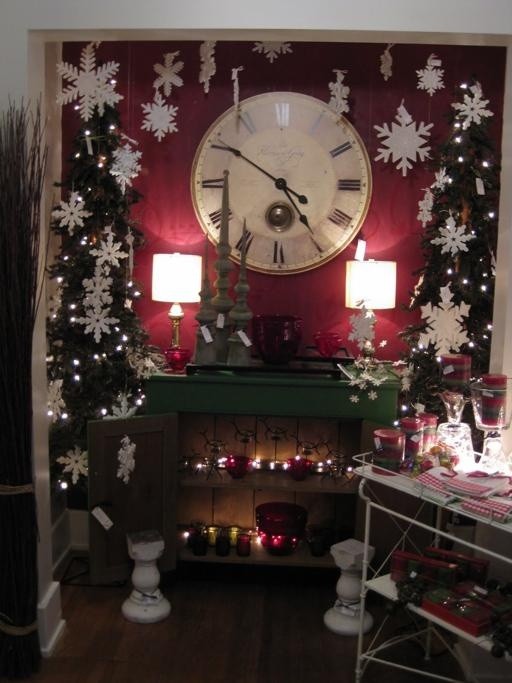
[314,333,342,358]
[224,455,252,478]
[287,458,312,481]
[163,350,192,374]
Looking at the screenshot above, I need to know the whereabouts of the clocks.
[190,91,372,276]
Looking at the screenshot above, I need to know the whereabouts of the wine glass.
[436,381,512,472]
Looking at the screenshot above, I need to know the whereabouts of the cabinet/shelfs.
[86,366,404,611]
[348,448,512,683]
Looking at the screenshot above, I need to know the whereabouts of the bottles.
[189,525,251,556]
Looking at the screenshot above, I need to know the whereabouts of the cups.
[310,537,324,558]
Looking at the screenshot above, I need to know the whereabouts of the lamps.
[150,251,203,370]
[344,258,397,372]
